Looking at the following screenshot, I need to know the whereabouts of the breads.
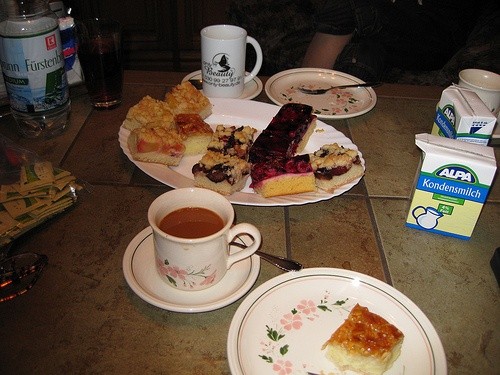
[127,122,186,167]
[123,94,174,131]
[164,80,214,120]
[172,112,214,157]
[320,302,405,375]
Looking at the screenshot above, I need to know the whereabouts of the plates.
[226,267,448,374]
[183,68,262,101]
[492,115,500,139]
[117,100,366,207]
[265,67,377,121]
[122,225,260,314]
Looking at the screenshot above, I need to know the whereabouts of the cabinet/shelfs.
[65,0,234,73]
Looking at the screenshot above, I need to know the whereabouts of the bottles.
[0,0,72,140]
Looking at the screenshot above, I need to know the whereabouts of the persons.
[302,0,481,68]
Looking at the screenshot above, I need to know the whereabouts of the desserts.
[191,150,253,195]
[249,103,317,164]
[249,152,316,197]
[208,125,256,162]
[309,142,364,193]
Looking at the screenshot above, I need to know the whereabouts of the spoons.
[189,78,203,90]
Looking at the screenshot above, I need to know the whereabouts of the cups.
[459,68,500,117]
[148,187,261,293]
[201,24,263,99]
[75,15,124,111]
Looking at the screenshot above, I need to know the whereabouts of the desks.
[0,70,499,373]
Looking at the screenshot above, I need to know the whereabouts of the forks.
[298,82,384,95]
